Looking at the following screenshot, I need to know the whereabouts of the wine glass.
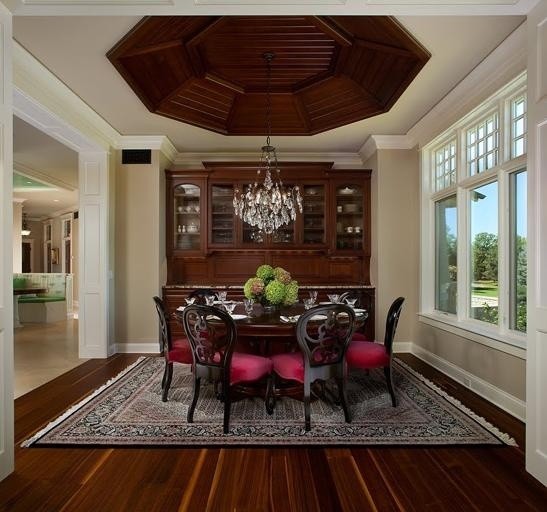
[183,292,254,318]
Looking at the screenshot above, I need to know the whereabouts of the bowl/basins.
[339,188,358,195]
[187,226,197,233]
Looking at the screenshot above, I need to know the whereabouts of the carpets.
[21,356,517,448]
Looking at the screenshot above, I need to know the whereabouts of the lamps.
[231,53,302,235]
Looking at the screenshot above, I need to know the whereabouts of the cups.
[348,226,361,233]
[303,291,358,314]
[337,206,342,213]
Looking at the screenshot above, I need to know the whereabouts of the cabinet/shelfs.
[165,178,370,262]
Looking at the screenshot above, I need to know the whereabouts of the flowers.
[244,265,299,306]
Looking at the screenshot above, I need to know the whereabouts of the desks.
[13,287,45,328]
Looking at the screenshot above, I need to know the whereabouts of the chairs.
[151,288,404,432]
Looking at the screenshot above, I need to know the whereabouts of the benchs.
[18,296,64,324]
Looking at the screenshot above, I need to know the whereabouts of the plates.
[176,235,192,249]
[294,309,367,320]
[344,204,360,211]
[176,306,186,311]
